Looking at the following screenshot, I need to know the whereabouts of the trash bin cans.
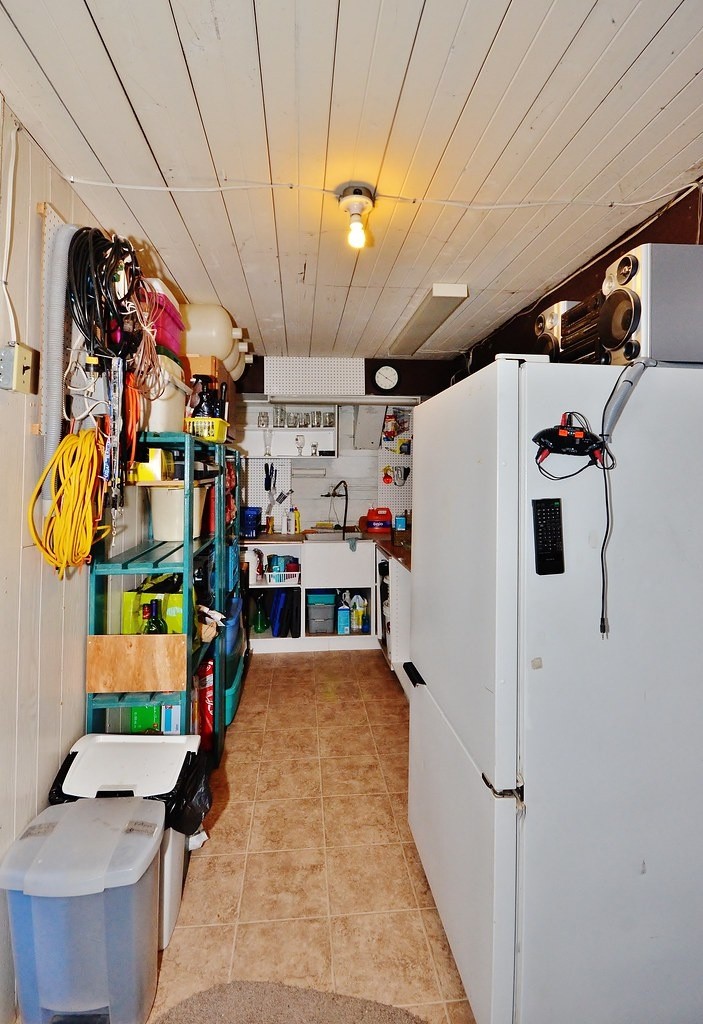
[0,798,166,1024]
[48,732,202,952]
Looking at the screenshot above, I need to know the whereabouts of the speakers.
[597,242,703,366]
[534,300,582,363]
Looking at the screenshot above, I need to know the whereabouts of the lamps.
[387,283,467,357]
[268,395,421,406]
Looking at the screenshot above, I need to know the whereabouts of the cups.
[311,411,321,427]
[263,429,272,456]
[273,405,286,427]
[288,412,298,427]
[287,563,298,579]
[323,412,335,427]
[298,413,310,427]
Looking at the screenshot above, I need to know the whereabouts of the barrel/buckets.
[383,601,390,661]
[240,551,258,583]
[149,485,211,542]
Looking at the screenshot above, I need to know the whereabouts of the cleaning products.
[366,501,392,532]
[281,506,300,535]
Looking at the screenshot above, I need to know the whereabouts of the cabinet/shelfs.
[232,401,339,457]
[376,548,414,699]
[240,544,377,651]
[82,433,242,768]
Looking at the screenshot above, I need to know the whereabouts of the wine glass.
[295,435,305,457]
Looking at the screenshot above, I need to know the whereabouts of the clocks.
[375,366,399,390]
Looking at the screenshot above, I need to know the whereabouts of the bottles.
[258,412,269,427]
[350,602,359,633]
[287,507,295,534]
[136,604,157,634]
[367,502,392,532]
[148,599,168,634]
[255,600,264,633]
[294,507,300,533]
[282,511,286,534]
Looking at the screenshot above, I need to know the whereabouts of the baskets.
[185,417,230,443]
[263,564,300,585]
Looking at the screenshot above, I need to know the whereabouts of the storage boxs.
[128,275,241,442]
[307,591,351,635]
[108,448,262,733]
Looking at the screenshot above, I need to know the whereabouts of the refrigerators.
[404,354,703,1024]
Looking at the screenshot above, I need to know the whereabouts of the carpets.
[154,979,428,1024]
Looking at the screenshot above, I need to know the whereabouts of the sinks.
[305,532,374,542]
